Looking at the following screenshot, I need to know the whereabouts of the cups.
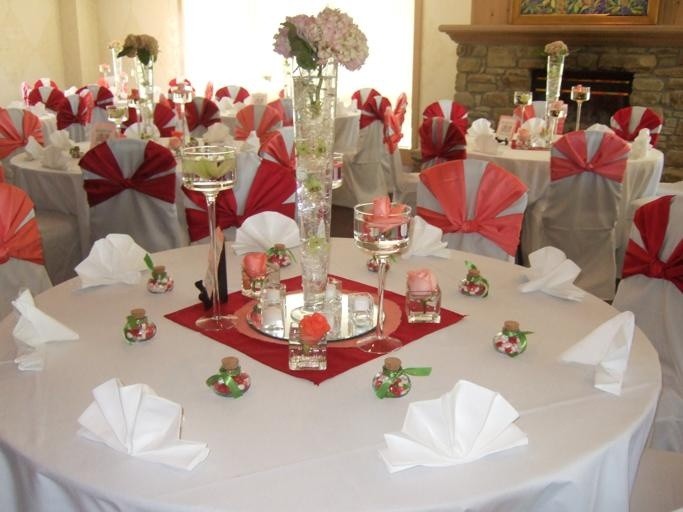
[405,282,443,325]
[330,151,344,190]
[496,115,513,145]
[346,290,374,329]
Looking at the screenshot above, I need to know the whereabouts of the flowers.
[272,7,370,109]
[544,40,569,64]
[99,34,159,81]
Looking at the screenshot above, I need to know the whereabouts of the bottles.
[264,244,293,268]
[371,356,411,397]
[256,260,285,333]
[72,146,82,158]
[457,266,490,299]
[493,321,526,356]
[146,265,175,294]
[209,355,251,399]
[122,308,157,343]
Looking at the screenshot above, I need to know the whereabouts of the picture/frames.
[511,0,661,25]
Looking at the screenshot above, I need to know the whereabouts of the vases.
[545,55,565,143]
[140,59,154,108]
[290,53,337,323]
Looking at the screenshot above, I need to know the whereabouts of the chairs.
[3,184,53,312]
[521,130,630,300]
[417,96,566,168]
[609,193,683,453]
[416,160,527,264]
[609,106,659,145]
[1,78,405,250]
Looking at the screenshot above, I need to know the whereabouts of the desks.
[1,238,662,506]
[466,134,662,216]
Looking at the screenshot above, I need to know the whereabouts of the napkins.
[556,310,635,396]
[378,379,529,472]
[71,233,154,287]
[74,378,208,470]
[232,211,300,255]
[519,247,584,301]
[11,289,78,370]
[402,216,451,261]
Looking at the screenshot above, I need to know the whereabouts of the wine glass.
[514,89,533,125]
[97,63,110,91]
[177,142,241,332]
[105,105,129,133]
[546,100,563,142]
[568,84,591,130]
[169,84,192,114]
[351,200,414,356]
